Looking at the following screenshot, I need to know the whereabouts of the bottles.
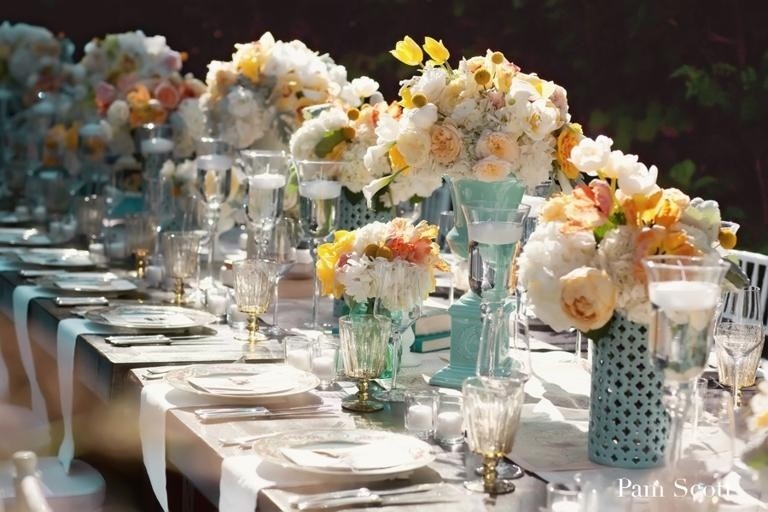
[424,179,451,225]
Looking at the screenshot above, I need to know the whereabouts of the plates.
[255,428,437,478]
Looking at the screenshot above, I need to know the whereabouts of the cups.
[397,200,422,224]
[432,395,466,446]
[545,469,721,512]
[404,390,439,440]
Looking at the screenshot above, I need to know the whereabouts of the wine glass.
[462,375,523,496]
[0,148,390,414]
[473,302,524,478]
[457,199,531,377]
[439,210,456,253]
[641,252,765,492]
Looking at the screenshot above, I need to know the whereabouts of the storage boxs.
[0,214,768,510]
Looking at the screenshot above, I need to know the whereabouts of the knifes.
[295,484,459,511]
